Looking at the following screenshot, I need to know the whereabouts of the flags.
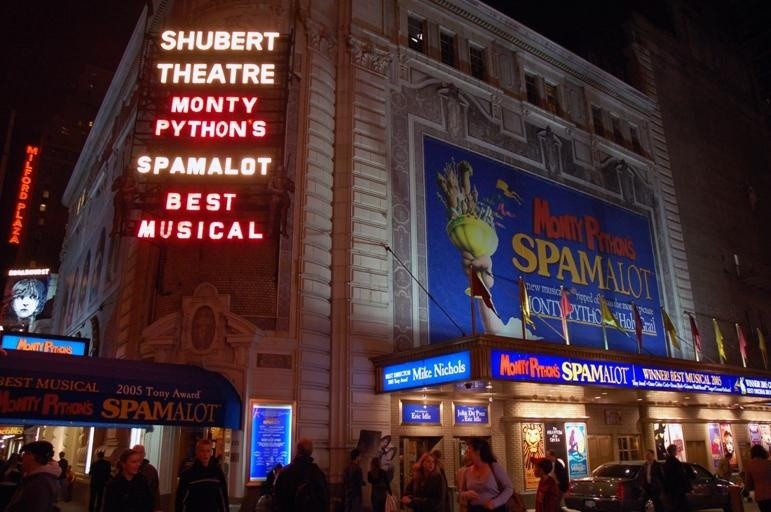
[470,266,499,319]
[602,297,628,338]
[690,316,701,354]
[519,281,537,332]
[714,323,726,360]
[758,328,768,368]
[737,325,748,365]
[633,306,643,347]
[560,290,574,343]
[663,309,679,350]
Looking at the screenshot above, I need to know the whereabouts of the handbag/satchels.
[384,495,399,512]
[488,462,527,512]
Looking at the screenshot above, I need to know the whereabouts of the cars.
[564,457,742,512]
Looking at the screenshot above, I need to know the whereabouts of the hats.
[530,457,552,475]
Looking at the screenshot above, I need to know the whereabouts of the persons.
[10,277,48,329]
